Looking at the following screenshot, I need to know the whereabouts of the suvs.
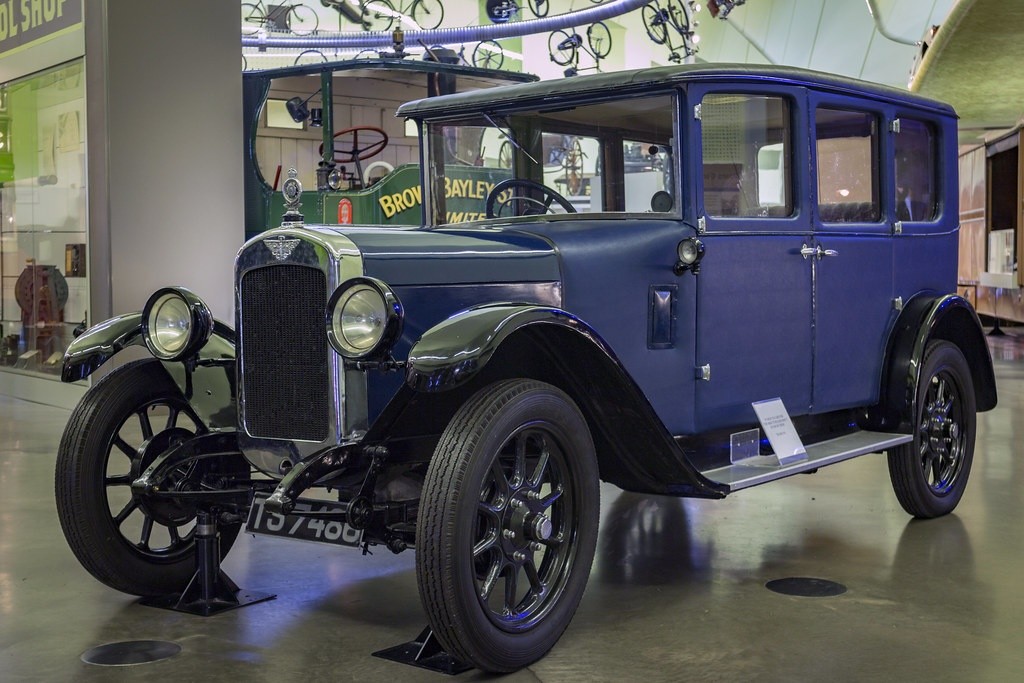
[50,56,999,675]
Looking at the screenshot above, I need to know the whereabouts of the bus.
[245,55,542,244]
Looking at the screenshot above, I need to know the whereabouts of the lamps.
[691,35,701,44]
[692,4,701,12]
[693,20,699,27]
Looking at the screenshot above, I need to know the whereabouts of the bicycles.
[496,131,591,196]
[242,0,319,38]
[547,21,613,77]
[641,1,701,65]
[428,39,504,68]
[487,0,549,23]
[360,0,444,36]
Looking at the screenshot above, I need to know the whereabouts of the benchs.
[744,199,933,222]
